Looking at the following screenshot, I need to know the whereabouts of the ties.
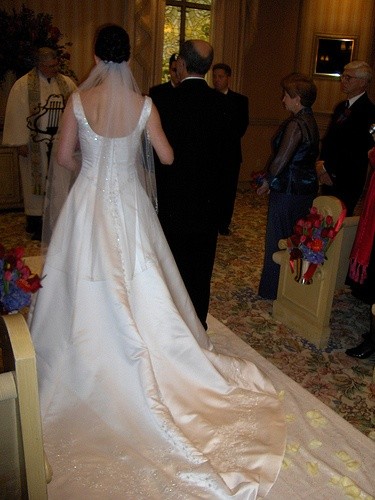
[345,100,349,109]
[47,78,51,83]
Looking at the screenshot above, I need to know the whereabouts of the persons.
[315,61,375,217]
[1,47,82,243]
[344,123,375,359]
[141,40,238,330]
[257,74,320,300]
[212,63,249,236]
[26,24,211,390]
[149,53,181,104]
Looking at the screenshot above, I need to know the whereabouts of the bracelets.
[322,164,327,173]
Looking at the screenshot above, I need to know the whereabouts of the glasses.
[341,74,362,81]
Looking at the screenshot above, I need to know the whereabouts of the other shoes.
[346,342,375,359]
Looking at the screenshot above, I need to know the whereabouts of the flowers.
[286,207,336,285]
[0,247,47,315]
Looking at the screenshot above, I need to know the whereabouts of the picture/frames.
[309,32,360,82]
